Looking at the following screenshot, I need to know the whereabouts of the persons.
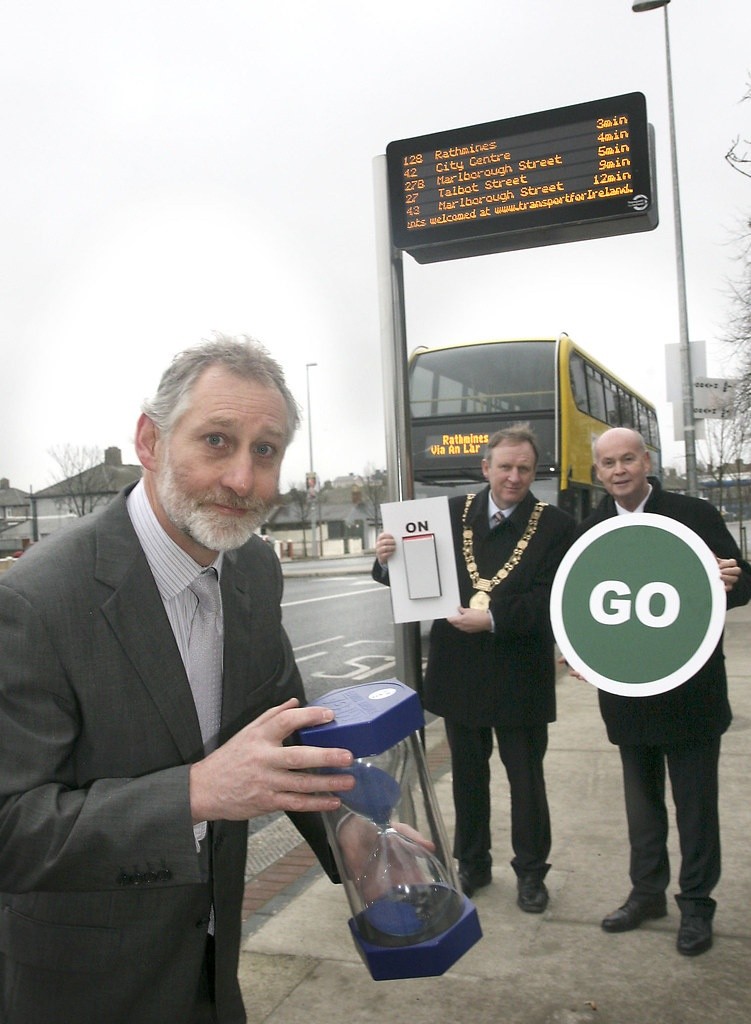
[372,428,573,915]
[0,338,436,1024]
[558,429,750,956]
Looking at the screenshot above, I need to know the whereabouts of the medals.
[458,494,547,612]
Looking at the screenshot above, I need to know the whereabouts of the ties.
[493,512,505,527]
[187,567,223,937]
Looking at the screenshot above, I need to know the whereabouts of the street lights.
[302,359,319,558]
[630,0,702,498]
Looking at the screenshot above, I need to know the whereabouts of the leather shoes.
[458,870,491,898]
[676,913,713,955]
[602,898,667,932]
[516,880,548,913]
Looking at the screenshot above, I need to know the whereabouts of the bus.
[405,329,662,502]
